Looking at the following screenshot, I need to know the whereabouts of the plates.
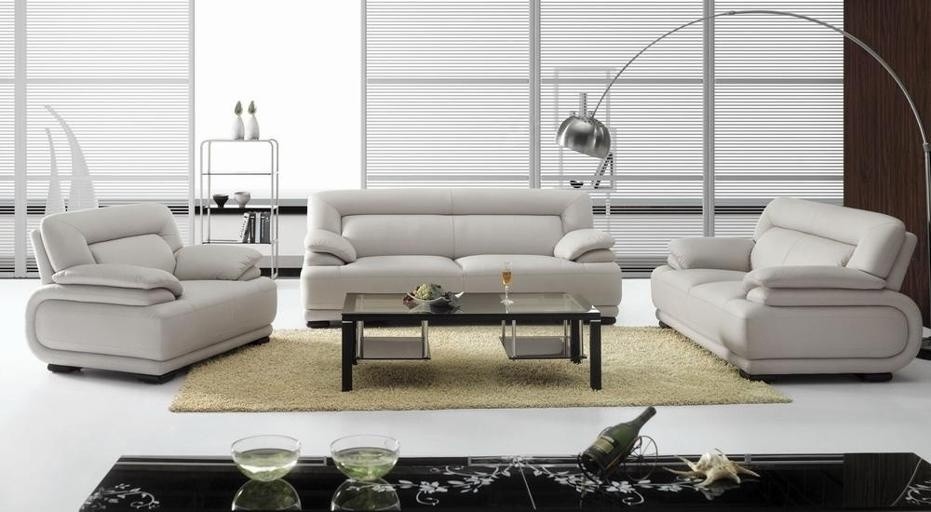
[408,303,464,315]
[406,290,465,303]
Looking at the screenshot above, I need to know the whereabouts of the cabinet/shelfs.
[199,138,279,279]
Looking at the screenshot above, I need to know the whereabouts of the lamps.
[556,8,931,227]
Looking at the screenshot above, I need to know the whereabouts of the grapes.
[412,284,432,300]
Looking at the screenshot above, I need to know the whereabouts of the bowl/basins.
[228,433,305,482]
[230,479,303,512]
[327,434,403,480]
[212,193,228,206]
[234,191,250,205]
[325,478,402,512]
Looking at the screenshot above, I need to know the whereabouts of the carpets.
[170,327,793,410]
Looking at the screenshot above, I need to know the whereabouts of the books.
[238,210,270,243]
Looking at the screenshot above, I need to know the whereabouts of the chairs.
[24,202,277,384]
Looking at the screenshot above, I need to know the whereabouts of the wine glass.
[499,262,514,305]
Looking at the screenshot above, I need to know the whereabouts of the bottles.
[581,406,656,476]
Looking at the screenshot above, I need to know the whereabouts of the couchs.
[301,188,621,330]
[650,197,923,382]
